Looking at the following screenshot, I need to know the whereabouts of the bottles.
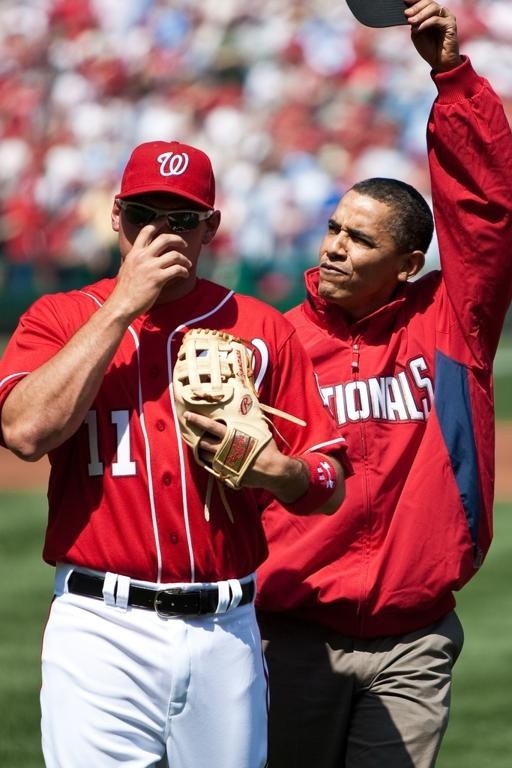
[114,140,217,213]
[344,0,414,28]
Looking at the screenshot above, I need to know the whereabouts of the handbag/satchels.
[272,452,338,516]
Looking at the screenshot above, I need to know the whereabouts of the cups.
[115,198,217,233]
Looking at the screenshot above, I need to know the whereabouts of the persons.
[251,0,512,768]
[1,141,355,768]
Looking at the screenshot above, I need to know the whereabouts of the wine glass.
[438,7,443,16]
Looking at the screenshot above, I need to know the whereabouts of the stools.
[65,567,256,620]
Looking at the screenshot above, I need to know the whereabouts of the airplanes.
[170,327,303,519]
